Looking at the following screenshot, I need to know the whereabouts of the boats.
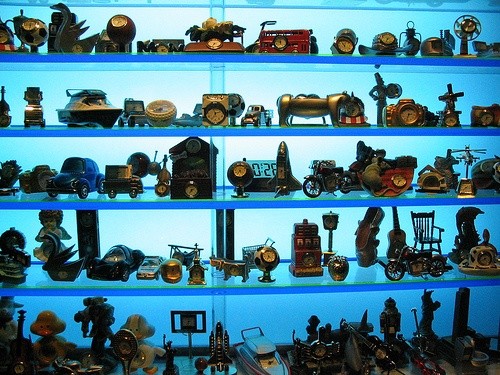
[232,326,291,375]
[57,88,124,129]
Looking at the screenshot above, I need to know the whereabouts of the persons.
[34,210,72,263]
[369,73,388,126]
[478,230,497,263]
[440,84,458,112]
[434,148,458,190]
[419,289,441,360]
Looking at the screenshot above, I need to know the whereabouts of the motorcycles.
[384,246,447,281]
[303,159,355,198]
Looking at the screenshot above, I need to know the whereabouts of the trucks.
[241,104,274,127]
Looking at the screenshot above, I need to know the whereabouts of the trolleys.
[242,237,276,265]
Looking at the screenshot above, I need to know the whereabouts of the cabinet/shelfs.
[0,0,500,375]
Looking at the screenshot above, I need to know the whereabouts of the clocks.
[458,244,500,276]
[258,28,311,54]
[254,246,280,282]
[381,98,427,126]
[336,90,371,127]
[330,28,358,54]
[12,10,49,53]
[169,137,219,199]
[357,150,417,197]
[454,14,483,58]
[470,103,500,127]
[184,38,245,52]
[358,31,414,56]
[202,93,230,127]
[288,211,339,278]
[226,158,255,198]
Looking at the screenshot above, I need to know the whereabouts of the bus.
[260,28,311,54]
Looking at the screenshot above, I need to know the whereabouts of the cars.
[86,245,146,282]
[104,164,144,198]
[136,255,166,282]
[45,157,105,200]
